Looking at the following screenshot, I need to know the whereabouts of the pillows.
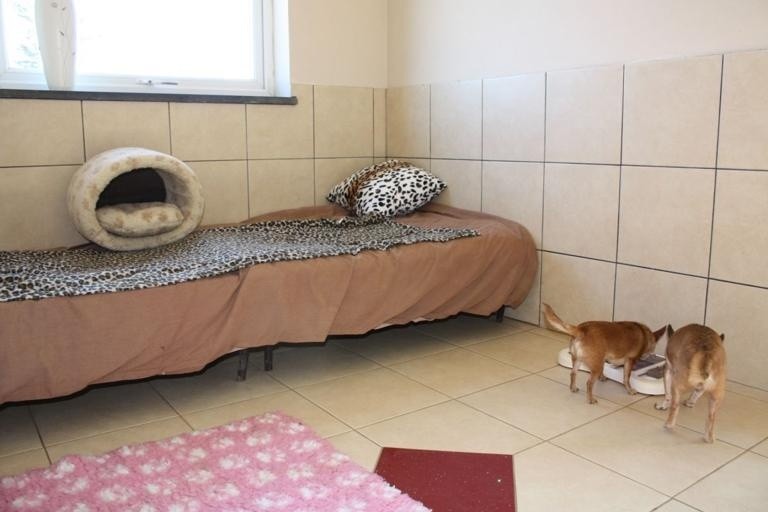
[324,159,446,218]
[100,200,184,236]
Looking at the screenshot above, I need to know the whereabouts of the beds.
[1,203,538,412]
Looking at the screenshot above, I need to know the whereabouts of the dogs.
[654,323,726,445]
[541,302,666,405]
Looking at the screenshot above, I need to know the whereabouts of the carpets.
[1,413,517,511]
